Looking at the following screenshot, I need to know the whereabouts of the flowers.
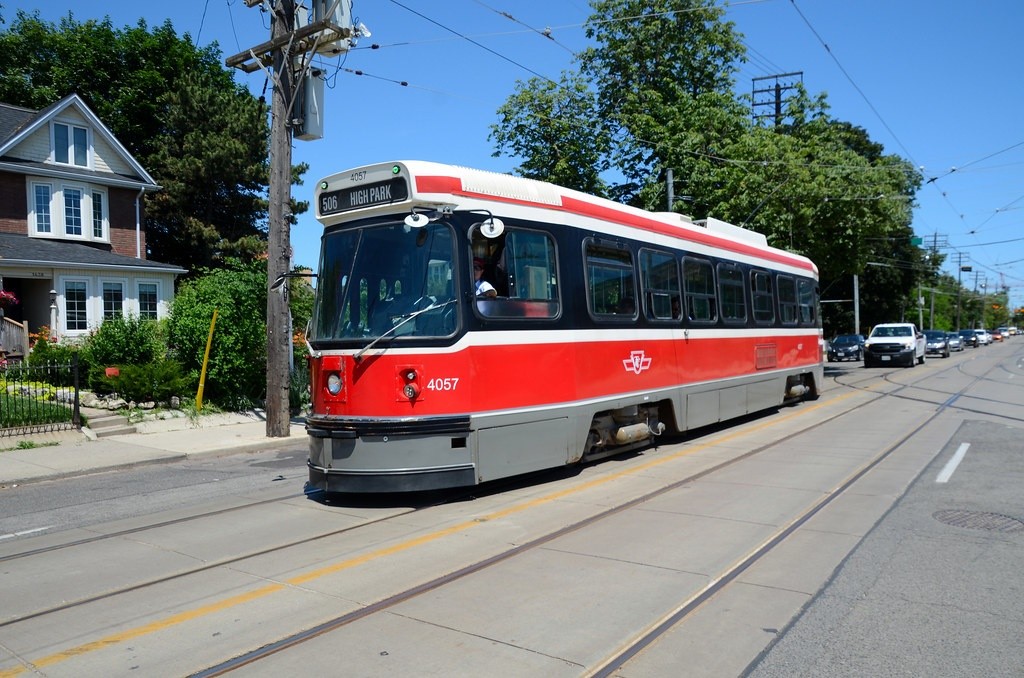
[0,290,19,307]
[29,326,57,344]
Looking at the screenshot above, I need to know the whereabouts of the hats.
[473,257,483,269]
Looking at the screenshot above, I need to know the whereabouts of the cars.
[975,329,988,346]
[987,326,1024,344]
[828,333,865,362]
[945,332,965,351]
[921,330,951,358]
[958,329,979,347]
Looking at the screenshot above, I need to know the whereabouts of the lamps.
[49,290,58,308]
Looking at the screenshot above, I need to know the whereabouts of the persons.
[473,257,497,297]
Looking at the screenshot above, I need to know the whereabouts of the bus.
[270,160,824,495]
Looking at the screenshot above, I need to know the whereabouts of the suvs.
[865,324,928,367]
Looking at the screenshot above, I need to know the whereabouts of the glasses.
[474,266,482,271]
